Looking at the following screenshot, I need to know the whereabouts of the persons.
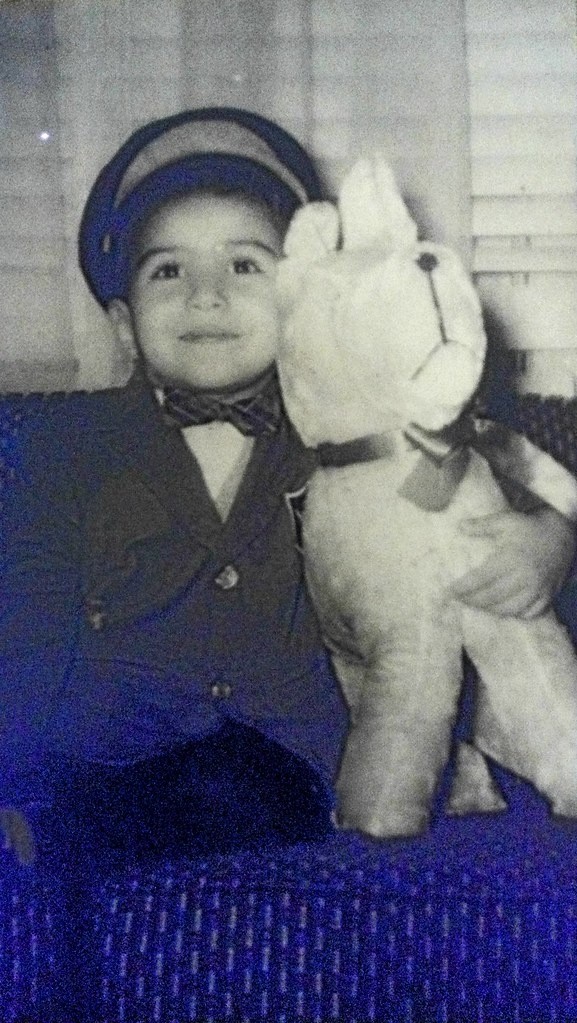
[0,109,577,865]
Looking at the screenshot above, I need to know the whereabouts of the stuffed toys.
[270,144,577,837]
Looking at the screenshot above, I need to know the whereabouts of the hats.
[76,107,324,313]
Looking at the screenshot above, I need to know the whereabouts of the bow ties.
[160,378,280,453]
